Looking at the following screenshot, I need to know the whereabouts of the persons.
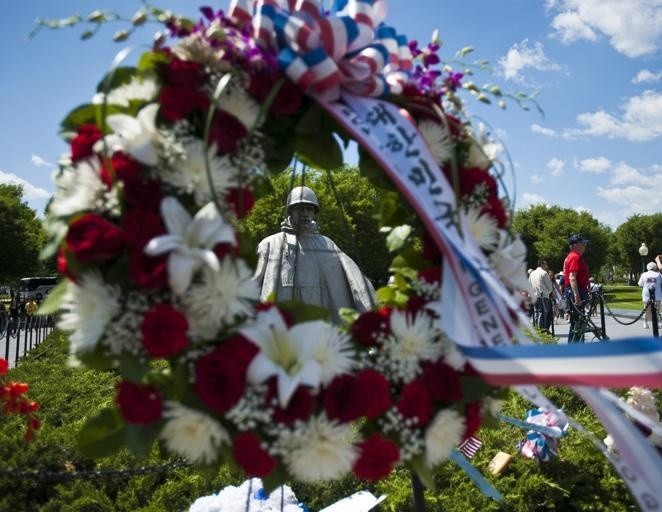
[251,186,380,326]
[24,298,39,331]
[527,234,662,345]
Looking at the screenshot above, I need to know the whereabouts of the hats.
[569,233,588,245]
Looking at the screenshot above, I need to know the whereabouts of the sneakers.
[658,323,662,329]
[644,323,649,329]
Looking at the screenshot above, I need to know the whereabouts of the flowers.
[28,0,522,487]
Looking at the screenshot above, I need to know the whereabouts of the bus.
[17,278,58,300]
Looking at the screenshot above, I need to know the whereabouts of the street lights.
[638,242,650,276]
